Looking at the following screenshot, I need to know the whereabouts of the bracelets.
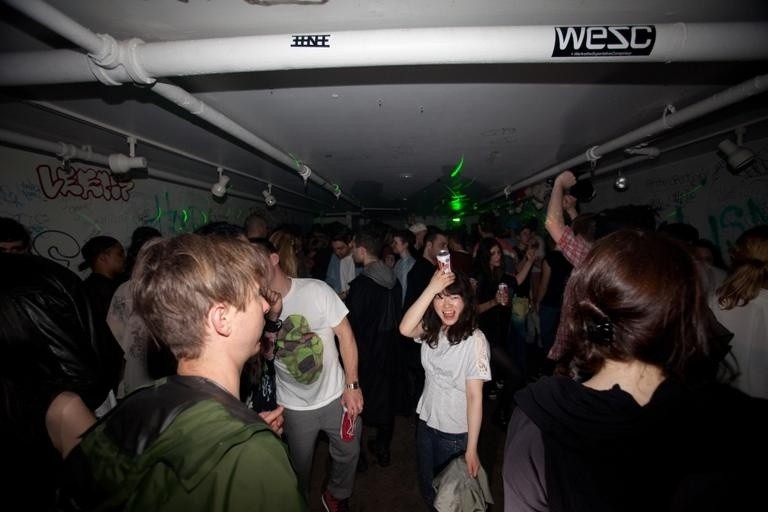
[265,318,282,333]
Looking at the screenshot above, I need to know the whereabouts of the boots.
[490,363,522,433]
[367,427,392,462]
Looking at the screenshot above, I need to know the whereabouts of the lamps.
[108,138,147,173]
[717,130,757,169]
[262,183,277,207]
[211,168,230,198]
[614,171,629,192]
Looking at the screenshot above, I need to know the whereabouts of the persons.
[0,215,31,253]
[248,238,365,512]
[0,342,97,512]
[473,236,536,431]
[324,234,364,304]
[345,230,403,475]
[447,234,471,267]
[542,171,608,374]
[410,223,427,251]
[501,228,768,512]
[243,215,268,239]
[56,231,305,512]
[239,242,284,413]
[668,224,727,290]
[0,250,118,418]
[474,213,513,257]
[269,231,298,278]
[510,225,535,340]
[400,229,449,438]
[390,230,417,308]
[77,236,127,390]
[698,237,727,268]
[697,222,768,399]
[397,263,492,512]
[535,243,574,358]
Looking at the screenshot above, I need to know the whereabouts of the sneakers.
[320,488,349,512]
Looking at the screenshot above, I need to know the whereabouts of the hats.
[76,234,119,271]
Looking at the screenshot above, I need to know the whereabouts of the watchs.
[344,381,361,390]
